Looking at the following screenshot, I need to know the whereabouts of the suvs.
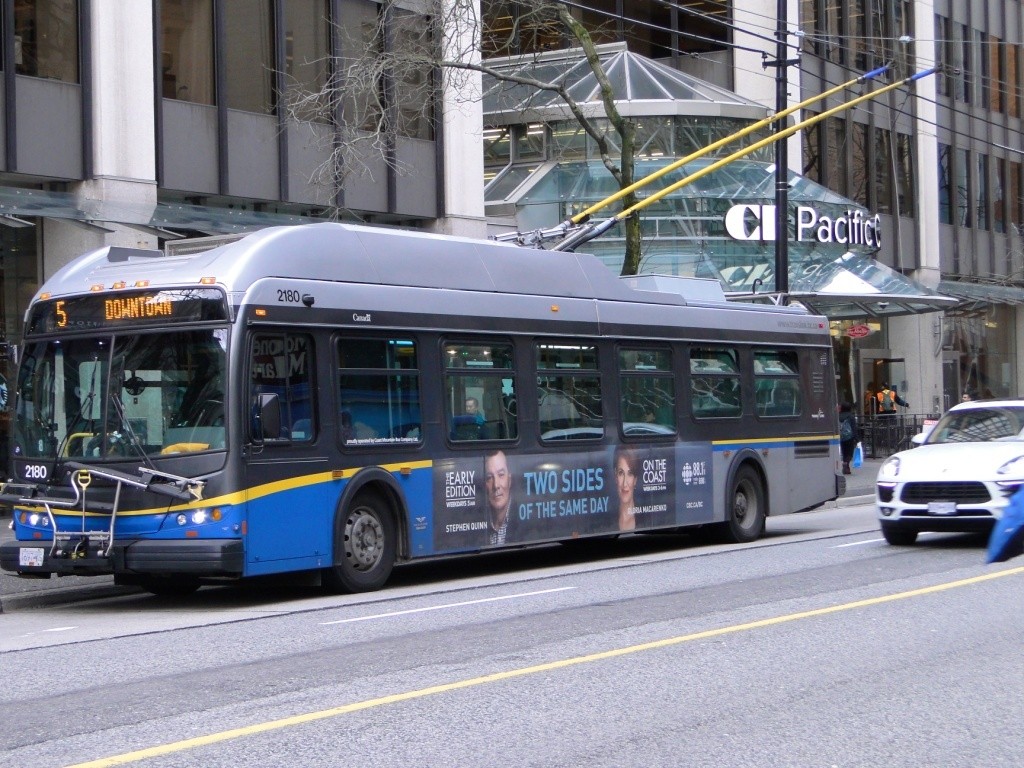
[876,397,1024,548]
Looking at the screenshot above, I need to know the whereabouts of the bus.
[1,58,948,602]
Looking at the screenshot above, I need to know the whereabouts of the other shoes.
[842,463,850,474]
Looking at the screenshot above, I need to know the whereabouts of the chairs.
[450,416,489,440]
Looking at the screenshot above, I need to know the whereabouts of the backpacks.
[879,389,894,412]
[839,417,853,442]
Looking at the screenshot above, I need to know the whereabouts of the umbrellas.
[987,483,1024,563]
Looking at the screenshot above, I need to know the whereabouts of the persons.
[644,405,657,423]
[875,382,908,425]
[467,449,523,545]
[466,398,484,423]
[839,403,859,474]
[864,382,874,414]
[962,393,972,401]
[588,445,651,532]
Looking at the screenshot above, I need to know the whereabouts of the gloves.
[906,402,909,407]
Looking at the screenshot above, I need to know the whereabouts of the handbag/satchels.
[852,441,864,467]
